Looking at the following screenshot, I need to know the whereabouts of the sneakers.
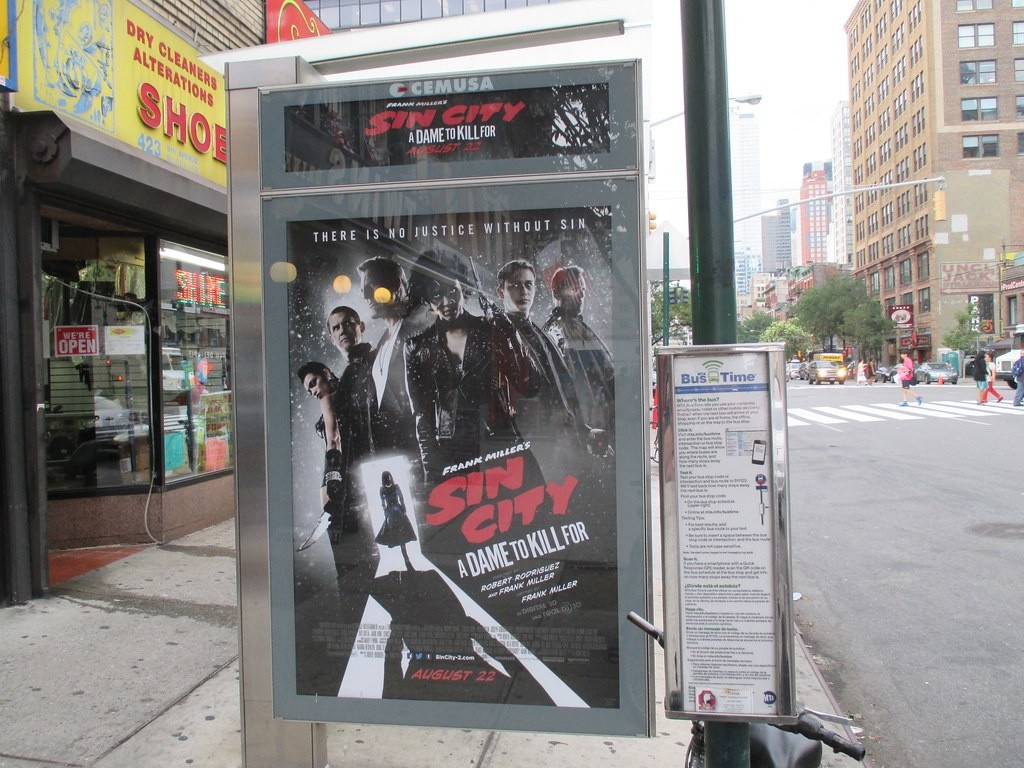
[917,396,923,405]
[900,402,908,406]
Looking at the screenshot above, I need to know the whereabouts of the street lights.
[646,95,766,409]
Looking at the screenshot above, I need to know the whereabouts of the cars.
[39,410,150,485]
[783,352,994,385]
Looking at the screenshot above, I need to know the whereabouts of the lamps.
[160,247,225,273]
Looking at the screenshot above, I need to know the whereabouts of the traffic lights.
[647,210,658,238]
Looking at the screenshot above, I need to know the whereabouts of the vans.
[160,344,197,405]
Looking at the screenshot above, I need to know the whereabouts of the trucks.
[993,323,1024,388]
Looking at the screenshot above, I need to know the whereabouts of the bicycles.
[627,607,870,768]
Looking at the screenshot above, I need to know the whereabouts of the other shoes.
[1013,402,1024,406]
[981,400,988,403]
[996,396,1004,403]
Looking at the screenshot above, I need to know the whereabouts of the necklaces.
[378,314,402,376]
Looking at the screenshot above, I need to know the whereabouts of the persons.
[897,350,923,408]
[537,265,617,564]
[480,258,585,561]
[844,356,880,389]
[325,303,416,578]
[401,242,553,562]
[358,255,441,583]
[371,469,422,577]
[1010,347,1024,407]
[972,349,989,404]
[296,358,386,633]
[981,351,1004,404]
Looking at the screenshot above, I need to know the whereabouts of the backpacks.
[909,372,919,386]
